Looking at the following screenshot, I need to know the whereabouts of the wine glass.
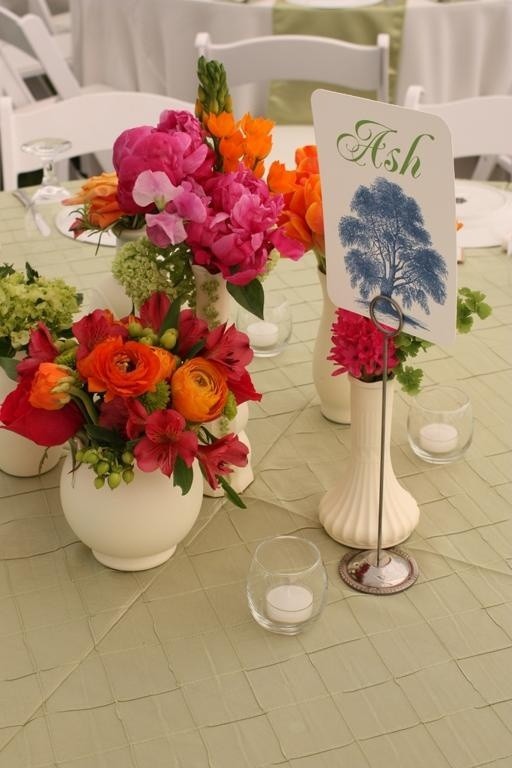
[21,138,73,240]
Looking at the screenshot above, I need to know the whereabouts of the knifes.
[13,192,52,238]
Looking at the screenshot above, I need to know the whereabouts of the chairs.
[0,5,120,174]
[195,31,391,176]
[0,87,188,185]
[404,83,511,264]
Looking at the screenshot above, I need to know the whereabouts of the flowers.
[265,144,461,278]
[61,50,303,331]
[1,289,251,513]
[327,280,490,400]
[0,263,87,372]
[62,169,152,256]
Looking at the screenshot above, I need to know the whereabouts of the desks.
[69,1,512,123]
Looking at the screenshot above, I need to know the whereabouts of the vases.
[203,403,255,498]
[112,226,145,255]
[313,267,354,426]
[189,263,234,329]
[1,368,59,480]
[58,444,204,574]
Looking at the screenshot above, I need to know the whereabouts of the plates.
[55,200,118,247]
[454,178,512,248]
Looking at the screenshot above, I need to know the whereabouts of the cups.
[245,537,328,636]
[237,292,292,358]
[406,384,475,465]
